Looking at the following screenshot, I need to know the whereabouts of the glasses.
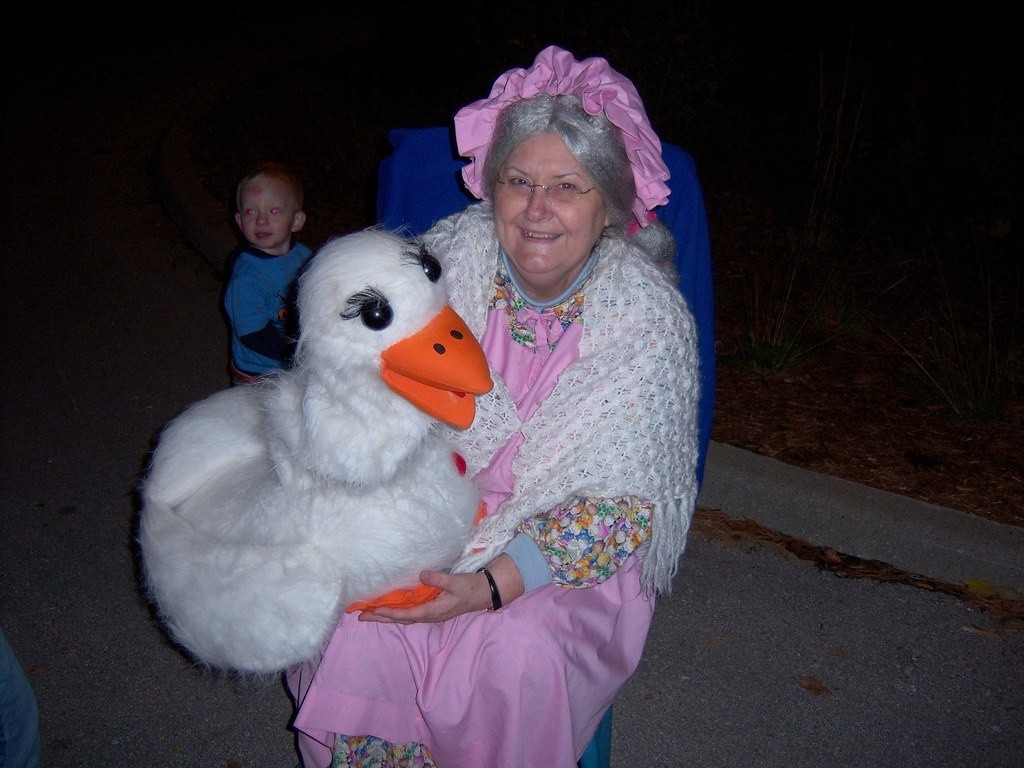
[496,176,596,202]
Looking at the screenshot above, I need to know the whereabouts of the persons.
[225,157,311,387]
[282,47,700,768]
[0,628,39,768]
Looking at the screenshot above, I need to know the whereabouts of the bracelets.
[476,567,502,611]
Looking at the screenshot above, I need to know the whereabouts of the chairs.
[376,123,715,768]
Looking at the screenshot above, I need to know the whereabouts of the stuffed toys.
[143,228,496,678]
